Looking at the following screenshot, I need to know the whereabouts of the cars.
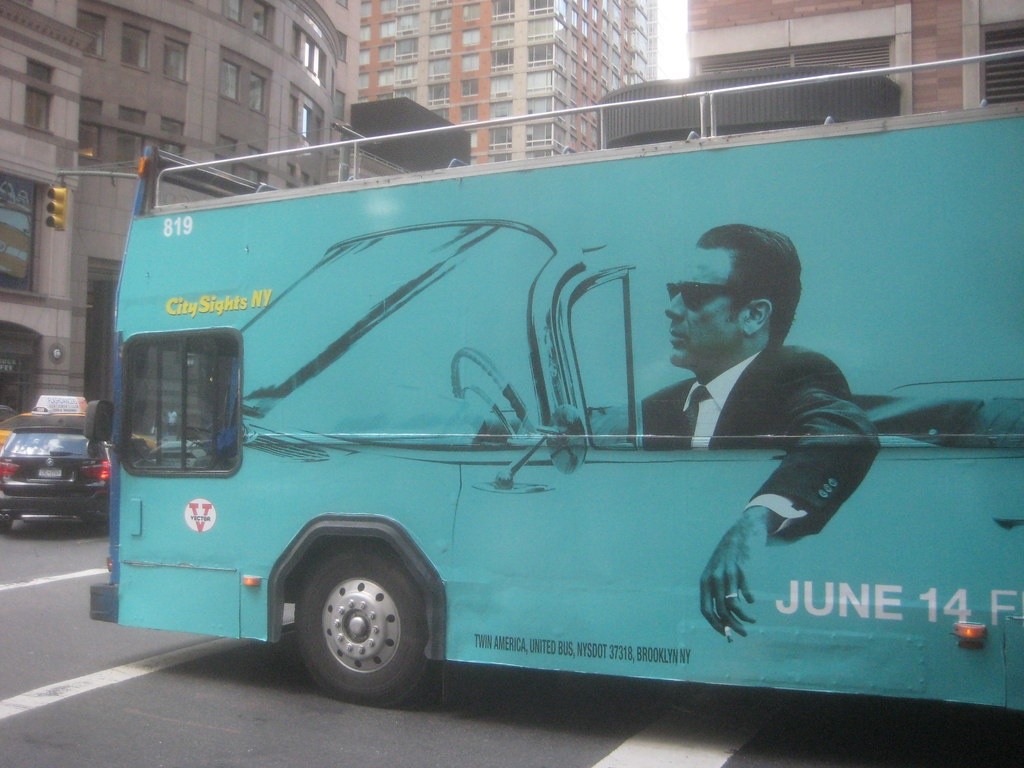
[0,404,19,422]
[131,433,157,451]
[0,394,158,452]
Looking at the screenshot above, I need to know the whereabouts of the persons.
[641,224,882,638]
[167,407,178,436]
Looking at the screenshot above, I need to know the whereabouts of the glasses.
[665,282,758,312]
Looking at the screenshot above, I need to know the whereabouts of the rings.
[725,593,738,598]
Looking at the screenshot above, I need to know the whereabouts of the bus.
[82,34,1024,734]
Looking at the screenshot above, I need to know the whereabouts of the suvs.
[0,424,110,533]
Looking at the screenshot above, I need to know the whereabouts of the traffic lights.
[45,188,65,231]
